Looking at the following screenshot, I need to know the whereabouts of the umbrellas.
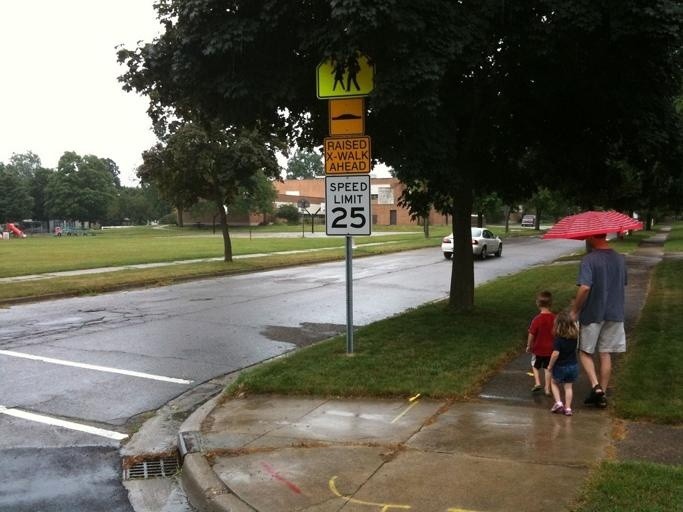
[542,210,644,240]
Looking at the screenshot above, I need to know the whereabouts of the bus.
[20,218,48,233]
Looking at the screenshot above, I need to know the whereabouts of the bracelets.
[526,344,530,346]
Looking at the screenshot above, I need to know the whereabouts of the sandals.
[583,385,607,408]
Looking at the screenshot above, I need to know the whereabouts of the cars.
[439,226,504,261]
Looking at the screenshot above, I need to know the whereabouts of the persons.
[568,233,628,407]
[547,308,578,416]
[525,291,559,396]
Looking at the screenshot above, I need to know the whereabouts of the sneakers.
[549,402,562,412]
[564,408,572,416]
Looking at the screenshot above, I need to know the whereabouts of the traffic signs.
[321,173,373,237]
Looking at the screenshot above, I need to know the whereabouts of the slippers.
[531,385,551,396]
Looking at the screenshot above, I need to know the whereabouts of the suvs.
[521,214,535,227]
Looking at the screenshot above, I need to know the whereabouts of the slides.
[8,224,26,238]
